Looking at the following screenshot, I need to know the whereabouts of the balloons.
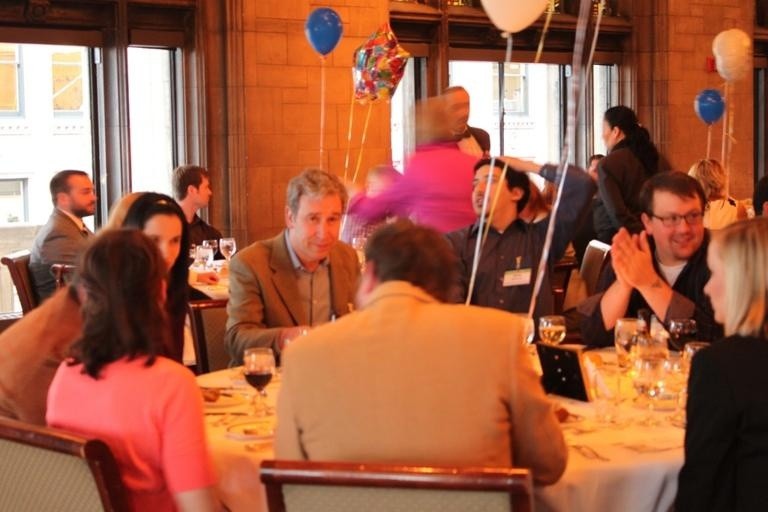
[353,24,409,105]
[478,1,551,35]
[304,7,342,57]
[694,89,724,125]
[711,29,752,82]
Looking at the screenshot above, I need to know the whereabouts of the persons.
[43,226,226,512]
[336,98,480,234]
[752,175,767,217]
[441,87,491,157]
[441,156,596,343]
[668,216,766,510]
[108,192,190,364]
[273,218,566,511]
[229,167,363,365]
[28,170,98,307]
[2,277,87,430]
[497,152,605,262]
[170,164,227,261]
[594,106,671,317]
[579,171,725,351]
[688,161,748,230]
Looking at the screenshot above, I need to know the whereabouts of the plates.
[221,421,276,442]
[203,390,242,407]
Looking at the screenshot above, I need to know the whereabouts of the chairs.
[0,415,128,512]
[260,458,537,512]
[188,299,235,375]
[1,250,38,316]
[556,238,613,339]
[49,262,76,289]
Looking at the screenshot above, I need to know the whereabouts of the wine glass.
[241,347,277,417]
[611,316,710,435]
[539,315,568,345]
[196,234,237,270]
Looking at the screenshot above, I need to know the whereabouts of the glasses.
[646,208,704,226]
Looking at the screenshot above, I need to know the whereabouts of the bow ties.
[453,129,473,142]
